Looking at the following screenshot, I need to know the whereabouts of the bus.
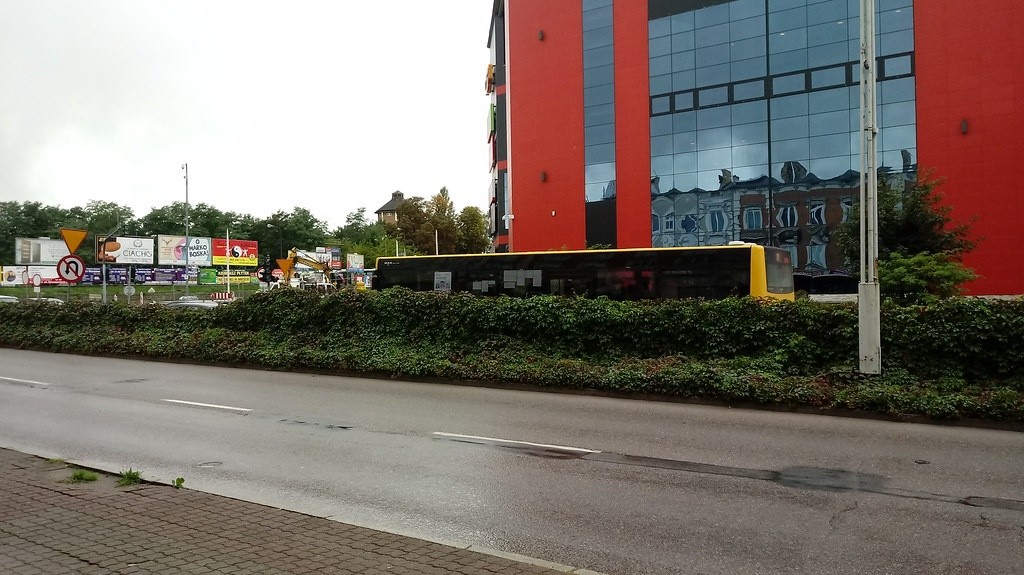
[329,268,375,293]
[371,238,795,302]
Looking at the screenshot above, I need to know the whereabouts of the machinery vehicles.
[276,246,367,293]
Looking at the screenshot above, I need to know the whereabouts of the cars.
[0,294,226,311]
[270,277,339,298]
